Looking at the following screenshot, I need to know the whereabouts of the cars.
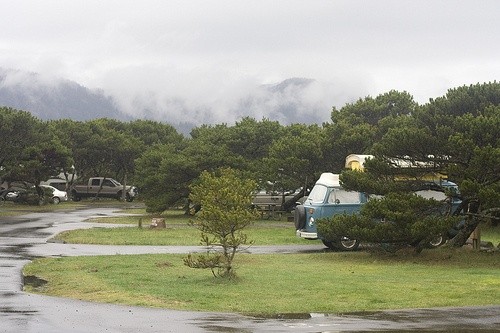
[6,185,61,206]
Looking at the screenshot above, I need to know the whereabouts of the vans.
[247,177,310,212]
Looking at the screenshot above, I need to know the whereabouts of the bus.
[294,154,467,252]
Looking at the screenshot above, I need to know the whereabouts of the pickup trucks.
[67,177,137,202]
